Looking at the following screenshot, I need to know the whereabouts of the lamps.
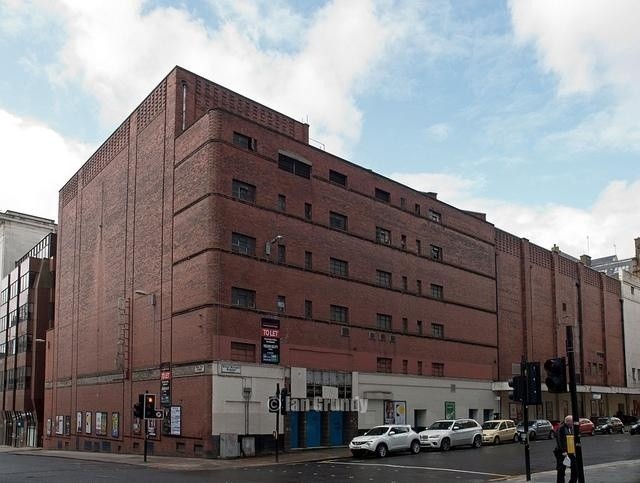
[264,234,283,255]
[34,338,50,350]
[133,289,157,307]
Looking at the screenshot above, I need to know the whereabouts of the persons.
[552,414,579,483]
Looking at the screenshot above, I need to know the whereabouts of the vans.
[348,415,640,458]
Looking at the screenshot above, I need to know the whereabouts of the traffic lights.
[145,393,155,418]
[133,393,145,419]
[544,358,567,392]
[507,375,524,402]
[280,387,287,415]
